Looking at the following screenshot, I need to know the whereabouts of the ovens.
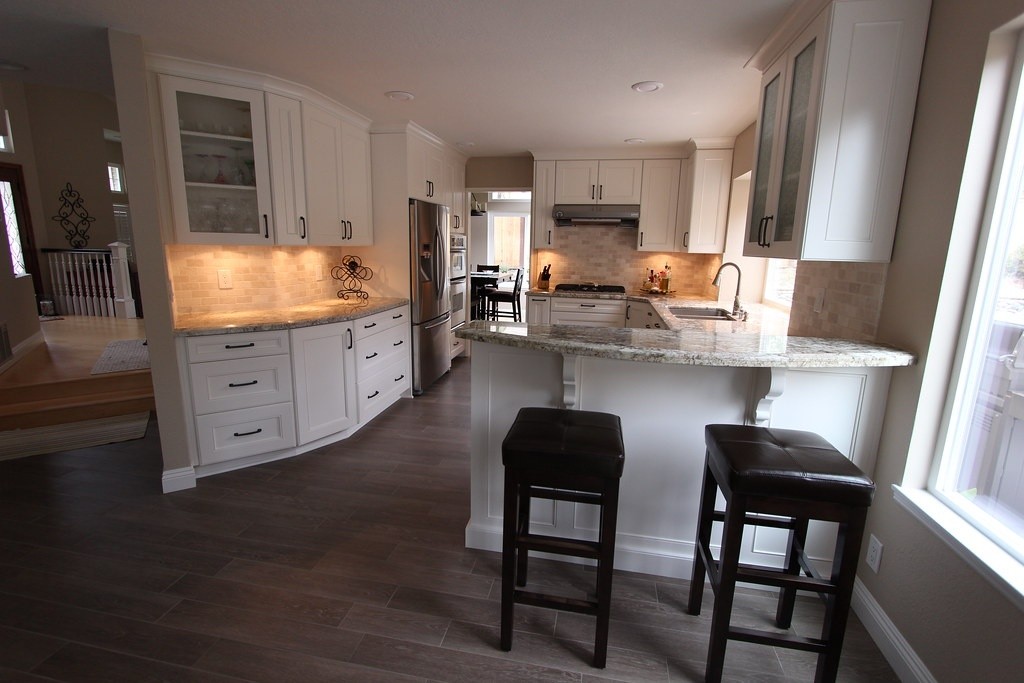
[449,249,466,280]
[449,278,465,328]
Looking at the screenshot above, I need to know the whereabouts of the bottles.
[644,266,672,291]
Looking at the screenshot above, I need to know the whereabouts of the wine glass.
[179,144,255,232]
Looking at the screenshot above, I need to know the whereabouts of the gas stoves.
[553,284,626,295]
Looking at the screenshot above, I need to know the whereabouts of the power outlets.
[813,286,826,314]
[316,264,323,280]
[218,270,232,289]
[864,532,882,573]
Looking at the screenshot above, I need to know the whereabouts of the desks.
[471,272,513,321]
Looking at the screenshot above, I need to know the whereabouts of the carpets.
[0,410,152,461]
[90,337,151,375]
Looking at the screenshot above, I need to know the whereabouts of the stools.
[494,408,626,670]
[687,424,872,683]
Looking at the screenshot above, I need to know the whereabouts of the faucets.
[711,261,742,315]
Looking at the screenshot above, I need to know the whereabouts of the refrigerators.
[409,200,451,396]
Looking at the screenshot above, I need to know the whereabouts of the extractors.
[552,205,639,229]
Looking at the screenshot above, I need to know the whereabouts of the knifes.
[538,264,551,281]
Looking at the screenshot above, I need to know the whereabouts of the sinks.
[674,315,736,320]
[667,306,732,315]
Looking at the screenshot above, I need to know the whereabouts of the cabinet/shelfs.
[554,160,644,204]
[265,91,308,247]
[531,159,555,250]
[626,301,650,329]
[677,149,733,253]
[299,98,372,246]
[741,0,933,259]
[526,295,552,323]
[291,322,358,443]
[353,304,413,429]
[159,75,271,240]
[549,298,627,327]
[637,160,682,251]
[444,153,470,233]
[639,307,667,330]
[188,333,294,463]
[407,133,445,204]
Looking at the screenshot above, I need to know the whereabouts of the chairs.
[473,264,523,322]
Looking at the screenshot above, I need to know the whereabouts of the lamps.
[470,193,487,216]
